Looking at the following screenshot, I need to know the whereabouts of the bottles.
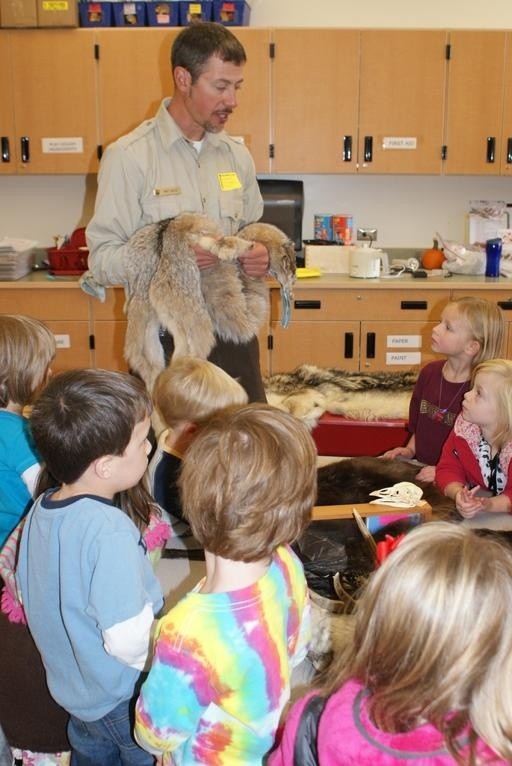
[350,244,388,278]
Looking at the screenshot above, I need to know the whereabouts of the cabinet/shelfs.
[256,281,512,375]
[0,29,98,177]
[0,284,133,418]
[272,29,448,173]
[443,28,512,177]
[97,26,271,176]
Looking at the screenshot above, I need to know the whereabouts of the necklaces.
[438,358,470,415]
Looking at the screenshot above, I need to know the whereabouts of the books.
[1,236,40,281]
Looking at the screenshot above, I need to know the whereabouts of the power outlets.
[357,226,377,242]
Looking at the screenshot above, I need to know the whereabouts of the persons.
[85,19,270,405]
[266,518,511,766]
[380,296,506,483]
[130,401,319,766]
[434,359,511,518]
[18,366,168,766]
[1,312,61,589]
[0,466,157,766]
[146,355,249,525]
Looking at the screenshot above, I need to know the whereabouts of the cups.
[484,238,503,278]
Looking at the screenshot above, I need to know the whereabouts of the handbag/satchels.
[441,241,490,277]
